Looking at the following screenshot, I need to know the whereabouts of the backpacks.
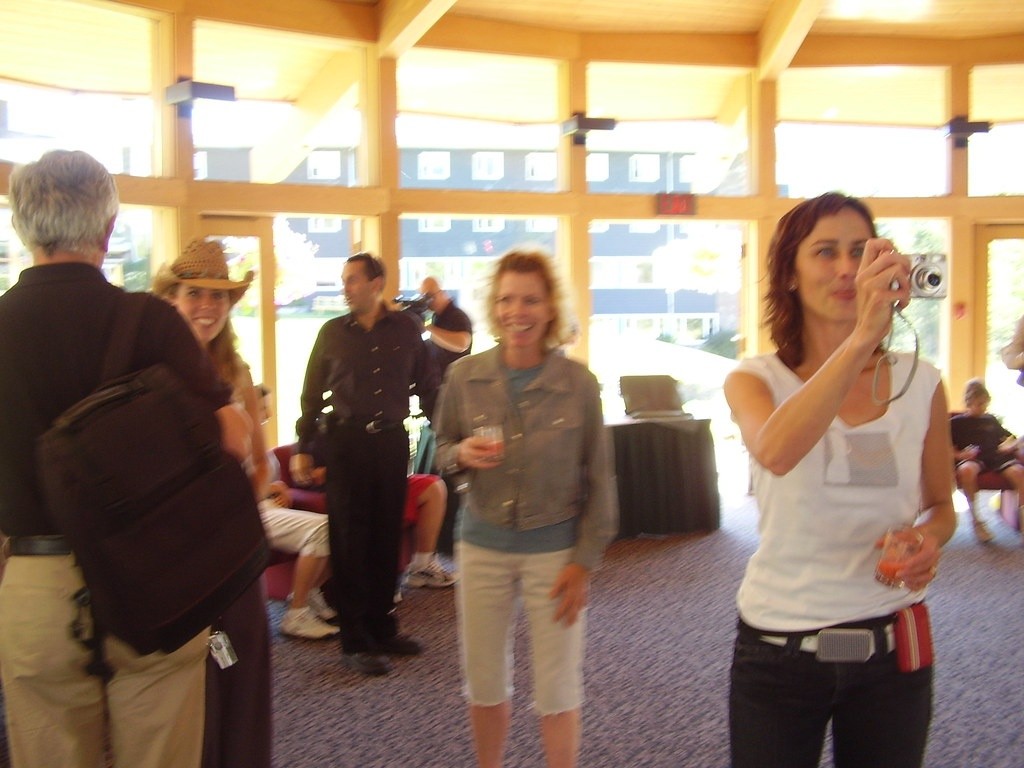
[37,289,272,658]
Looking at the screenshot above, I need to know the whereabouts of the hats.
[964,381,991,408]
[151,240,253,308]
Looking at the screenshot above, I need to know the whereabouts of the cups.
[473,426,504,461]
[873,527,923,589]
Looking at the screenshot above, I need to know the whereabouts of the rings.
[930,567,937,578]
[916,532,923,544]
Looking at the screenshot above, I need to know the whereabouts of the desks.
[608,418,721,543]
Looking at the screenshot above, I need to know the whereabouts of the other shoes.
[974,520,994,545]
[349,643,393,674]
[379,628,420,657]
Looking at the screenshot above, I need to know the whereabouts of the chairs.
[263,443,414,602]
[618,375,692,420]
[948,411,1020,533]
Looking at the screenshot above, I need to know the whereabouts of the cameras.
[889,252,947,297]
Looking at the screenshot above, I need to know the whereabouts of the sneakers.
[287,588,337,620]
[281,605,340,639]
[407,554,459,587]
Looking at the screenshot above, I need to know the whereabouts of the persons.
[293,407,460,601]
[1002,313,1024,387]
[289,253,438,676]
[721,192,957,768]
[236,385,339,641]
[412,277,473,424]
[0,149,251,768]
[432,251,618,768]
[151,239,271,767]
[948,377,1024,543]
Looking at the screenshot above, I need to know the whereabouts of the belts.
[756,623,896,663]
